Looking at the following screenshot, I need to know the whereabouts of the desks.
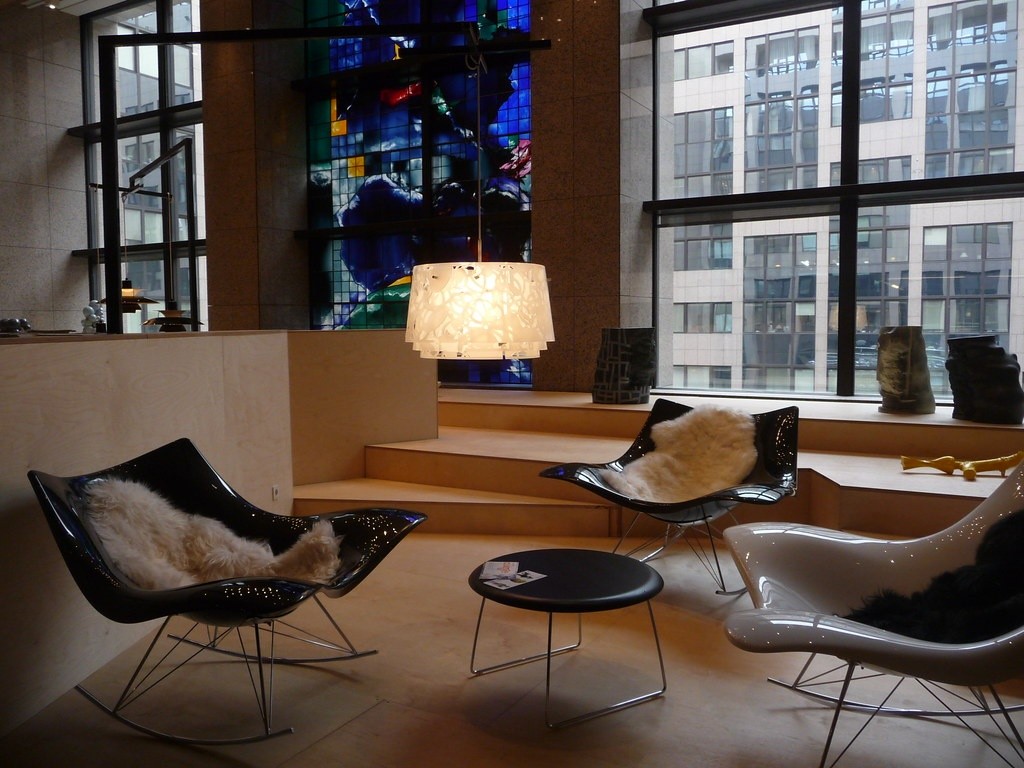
[466,548,668,731]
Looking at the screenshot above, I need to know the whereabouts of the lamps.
[97,193,159,313]
[139,194,205,332]
[403,60,556,360]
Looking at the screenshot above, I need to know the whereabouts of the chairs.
[722,462,1023,768]
[537,397,800,597]
[28,437,428,746]
